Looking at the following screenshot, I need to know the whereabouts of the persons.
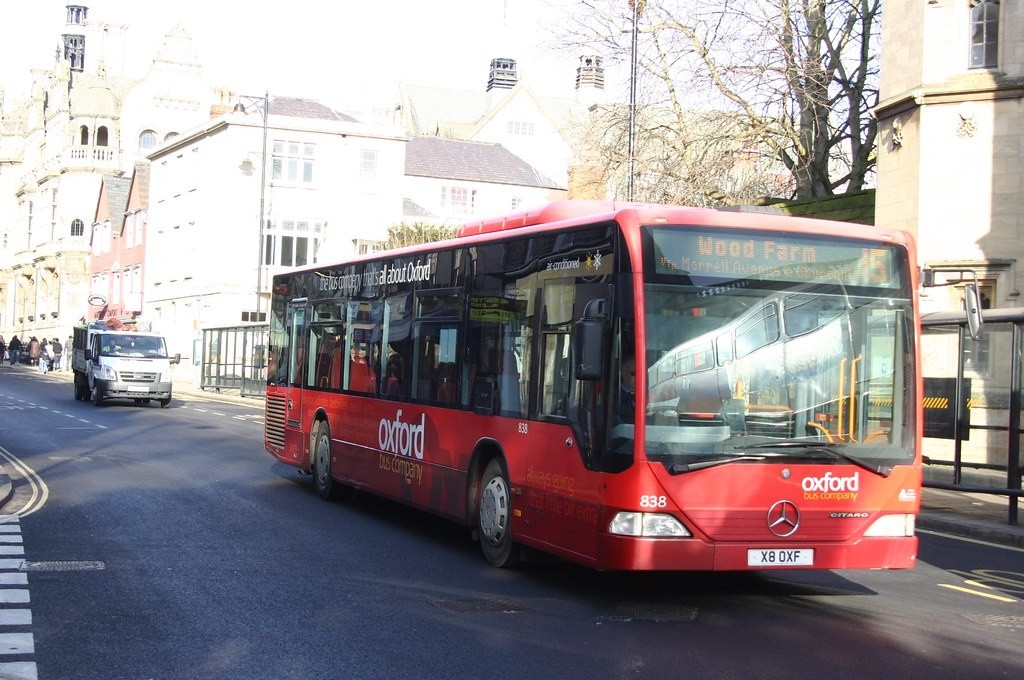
[103,337,121,353]
[0,334,74,375]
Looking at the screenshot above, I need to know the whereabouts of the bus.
[263,200,984,590]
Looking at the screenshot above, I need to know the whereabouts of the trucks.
[71,320,181,408]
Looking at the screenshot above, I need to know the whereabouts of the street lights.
[228,91,270,323]
